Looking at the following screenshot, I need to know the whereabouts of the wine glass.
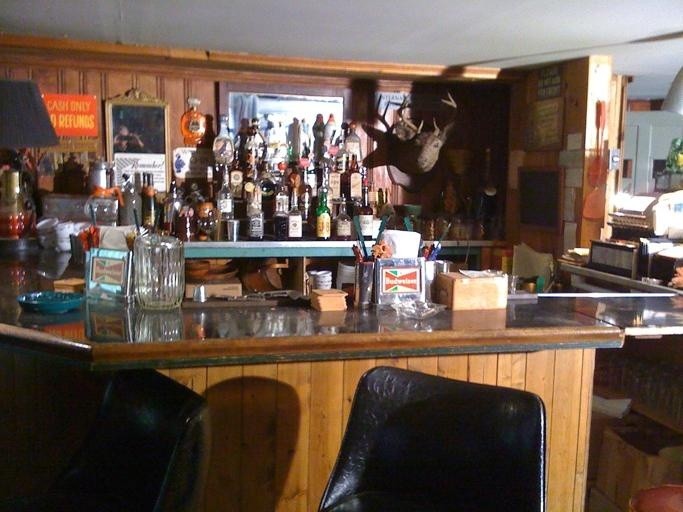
[197,208,219,242]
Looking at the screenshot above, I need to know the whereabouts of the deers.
[394,89,460,177]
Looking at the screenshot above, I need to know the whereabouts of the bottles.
[211,113,373,241]
[180,97,208,145]
[223,220,239,241]
[134,205,185,311]
[88,155,114,193]
[377,188,384,214]
[143,174,156,229]
[163,177,182,233]
[382,188,394,216]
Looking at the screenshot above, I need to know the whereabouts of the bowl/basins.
[403,205,421,218]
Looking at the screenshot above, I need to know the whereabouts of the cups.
[36,216,74,252]
[418,260,449,305]
[507,274,517,295]
[177,216,195,238]
[353,260,374,308]
[315,269,331,289]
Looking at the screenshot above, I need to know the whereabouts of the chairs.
[19,367,212,512]
[316,366,549,511]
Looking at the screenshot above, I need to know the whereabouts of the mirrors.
[103,87,173,196]
[213,78,355,151]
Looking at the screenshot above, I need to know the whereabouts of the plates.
[187,264,236,280]
[17,312,86,326]
[15,290,85,314]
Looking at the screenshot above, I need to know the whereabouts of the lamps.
[1,80,60,189]
